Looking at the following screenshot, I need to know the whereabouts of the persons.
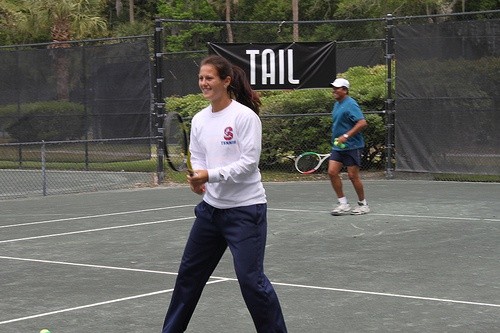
[161,56,288,333]
[327,78,371,217]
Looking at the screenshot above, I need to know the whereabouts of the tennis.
[40,328,51,333]
[334,138,347,148]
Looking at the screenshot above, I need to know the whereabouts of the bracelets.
[343,134,351,141]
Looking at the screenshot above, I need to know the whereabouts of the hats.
[330,78,350,89]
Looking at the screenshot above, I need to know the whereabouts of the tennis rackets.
[295,151,332,174]
[163,111,205,193]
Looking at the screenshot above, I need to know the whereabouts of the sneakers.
[351,205,370,215]
[330,203,352,215]
